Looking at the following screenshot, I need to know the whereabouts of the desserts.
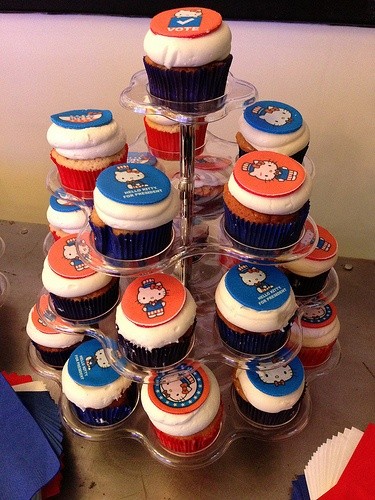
[27,94,341,457]
[142,7,233,111]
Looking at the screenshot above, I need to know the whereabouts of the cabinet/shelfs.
[24,66,342,470]
